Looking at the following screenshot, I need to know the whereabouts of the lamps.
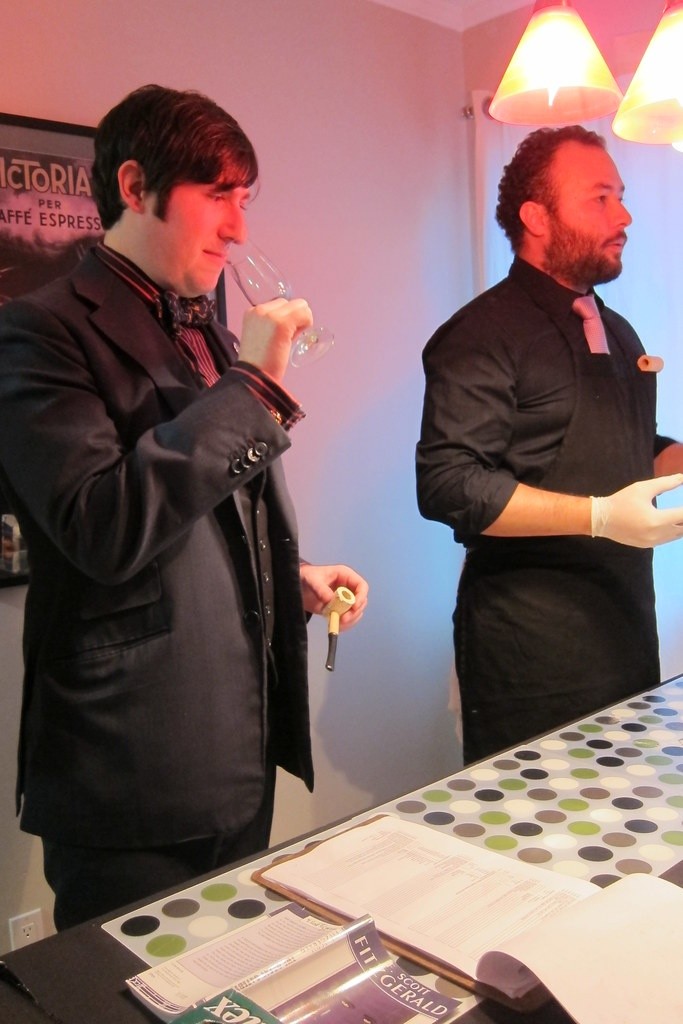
[612,0,683,152]
[488,0,623,126]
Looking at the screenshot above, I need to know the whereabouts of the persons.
[412,125,683,767]
[0,84,369,933]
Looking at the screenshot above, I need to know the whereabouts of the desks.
[0,673,683,1024]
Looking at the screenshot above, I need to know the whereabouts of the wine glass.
[227,236,336,367]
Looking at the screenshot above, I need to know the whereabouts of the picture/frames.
[0,111,109,587]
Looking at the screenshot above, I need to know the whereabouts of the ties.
[573,296,610,354]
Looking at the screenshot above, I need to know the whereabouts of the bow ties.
[164,289,218,338]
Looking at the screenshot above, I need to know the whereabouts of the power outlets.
[9,908,43,951]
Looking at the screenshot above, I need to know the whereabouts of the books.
[125,901,462,1024]
